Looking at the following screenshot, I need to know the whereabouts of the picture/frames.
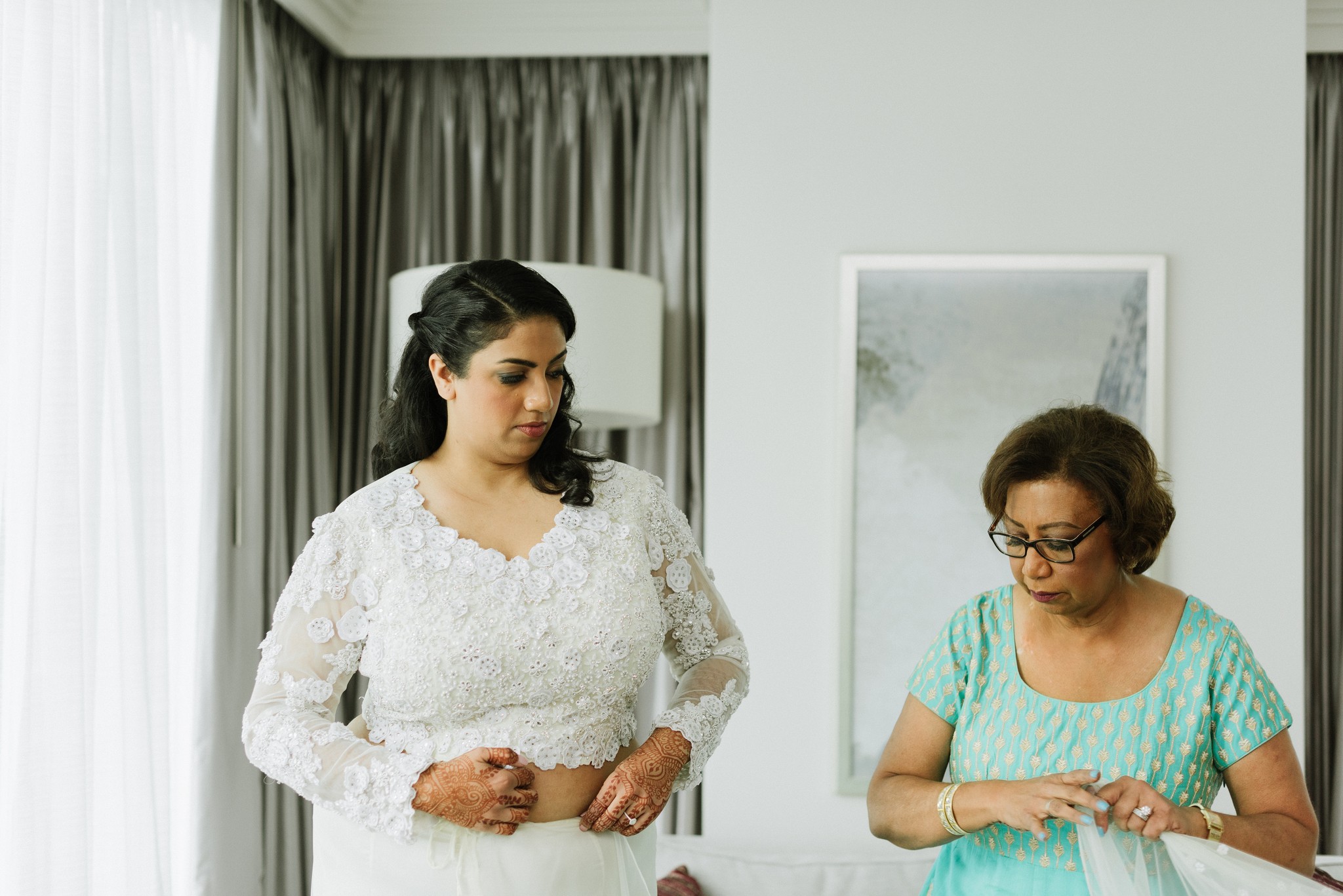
[835,253,1170,797]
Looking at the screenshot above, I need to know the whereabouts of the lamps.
[388,260,663,431]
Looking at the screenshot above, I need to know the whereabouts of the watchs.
[1188,801,1225,843]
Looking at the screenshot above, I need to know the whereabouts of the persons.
[235,258,753,896]
[867,402,1323,896]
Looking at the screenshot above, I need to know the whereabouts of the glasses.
[988,504,1109,563]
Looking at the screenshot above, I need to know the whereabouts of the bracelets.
[944,781,972,838]
[936,783,965,836]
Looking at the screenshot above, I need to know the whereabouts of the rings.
[1136,806,1152,816]
[1131,808,1149,822]
[1044,798,1056,816]
[625,812,639,827]
